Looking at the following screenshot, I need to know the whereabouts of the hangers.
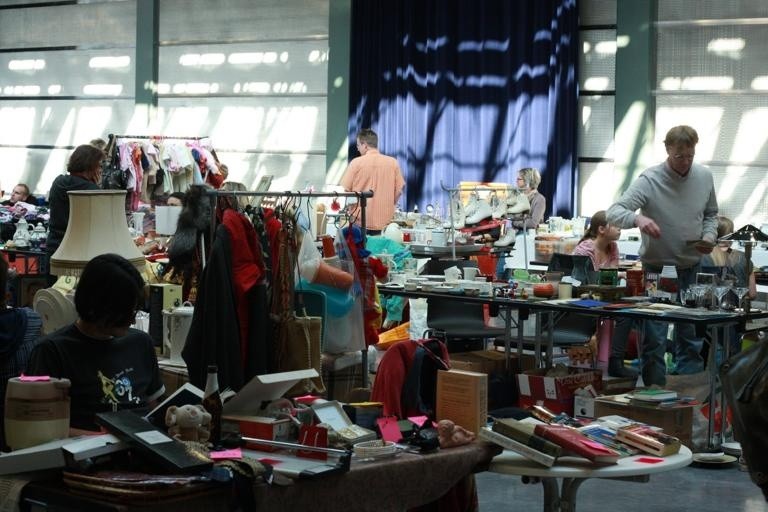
[116,135,201,145]
[227,189,365,231]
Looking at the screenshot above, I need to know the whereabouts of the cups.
[379,253,507,297]
[300,259,354,289]
[625,269,644,296]
[538,216,586,234]
[545,271,560,295]
[160,298,196,368]
[597,268,619,299]
[558,283,572,299]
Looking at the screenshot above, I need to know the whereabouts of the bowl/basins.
[720,442,741,457]
[533,284,554,298]
[354,440,396,456]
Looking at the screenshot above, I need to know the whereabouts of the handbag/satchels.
[267,317,326,393]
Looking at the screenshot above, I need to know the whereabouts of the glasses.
[672,154,694,160]
[516,178,523,180]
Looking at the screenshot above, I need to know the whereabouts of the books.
[473,413,681,471]
[625,386,679,403]
[597,389,638,407]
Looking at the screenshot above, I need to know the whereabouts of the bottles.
[146,219,161,240]
[402,227,448,247]
[201,366,222,444]
[133,231,144,248]
[659,265,678,291]
[130,211,146,238]
[570,255,593,285]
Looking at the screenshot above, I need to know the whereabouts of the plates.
[692,452,737,464]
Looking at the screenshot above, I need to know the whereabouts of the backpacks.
[369,339,451,419]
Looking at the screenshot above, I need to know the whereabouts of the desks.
[1,421,478,511]
[478,420,695,512]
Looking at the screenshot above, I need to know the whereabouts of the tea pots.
[12,215,47,250]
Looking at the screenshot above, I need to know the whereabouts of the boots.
[609,357,637,376]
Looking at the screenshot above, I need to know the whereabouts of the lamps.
[51,190,146,278]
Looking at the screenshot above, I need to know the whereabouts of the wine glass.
[681,283,750,312]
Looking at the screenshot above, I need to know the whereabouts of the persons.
[698,213,757,373]
[506,168,547,233]
[43,144,103,288]
[9,182,30,204]
[23,252,169,439]
[334,128,405,238]
[218,180,249,209]
[602,123,721,393]
[570,208,642,382]
[165,191,188,207]
[0,256,52,397]
